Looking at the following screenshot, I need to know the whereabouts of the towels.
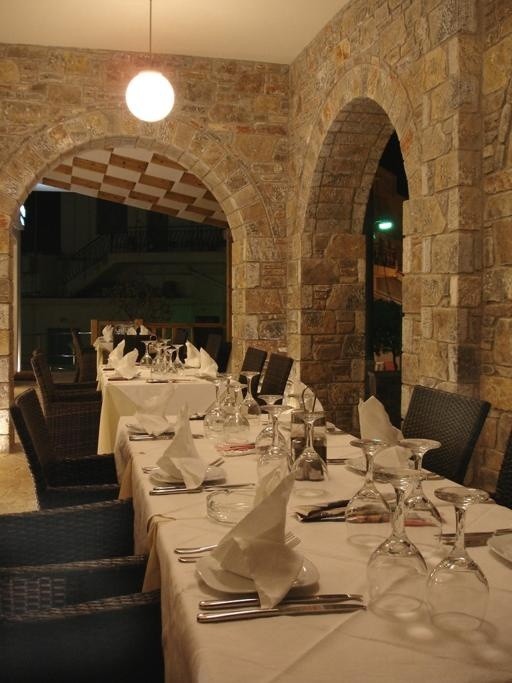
[139,325,148,335]
[102,324,114,342]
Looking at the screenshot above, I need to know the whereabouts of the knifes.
[149,481,252,497]
[127,434,204,441]
[191,594,367,623]
[433,526,512,547]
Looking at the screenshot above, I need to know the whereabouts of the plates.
[486,532,510,560]
[152,464,230,482]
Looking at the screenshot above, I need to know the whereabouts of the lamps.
[125,0,175,124]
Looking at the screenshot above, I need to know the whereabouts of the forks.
[171,524,304,563]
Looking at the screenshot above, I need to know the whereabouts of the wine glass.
[113,324,146,335]
[196,372,329,505]
[141,338,186,381]
[344,439,491,632]
